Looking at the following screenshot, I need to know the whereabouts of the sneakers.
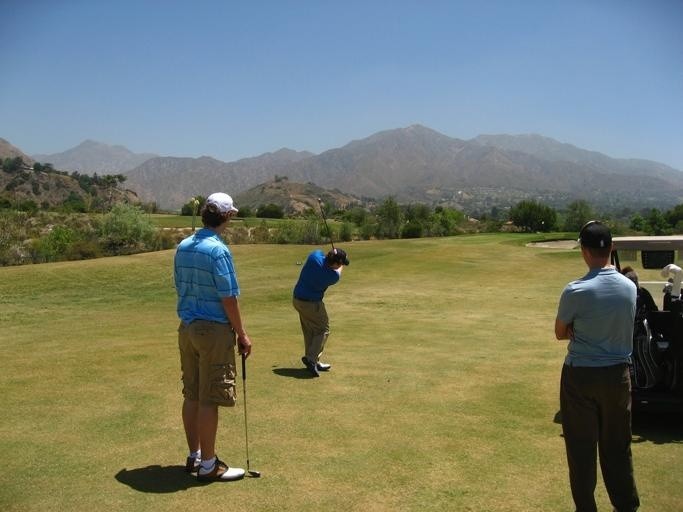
[197,456,246,481]
[185,457,201,479]
[302,357,320,377]
[317,361,331,369]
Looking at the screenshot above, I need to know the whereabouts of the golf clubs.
[317,198,335,249]
[238,342,261,477]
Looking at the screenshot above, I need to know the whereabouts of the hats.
[206,193,239,213]
[328,248,349,265]
[577,220,611,248]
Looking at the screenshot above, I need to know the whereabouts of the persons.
[173,191,251,481]
[555,223,640,512]
[292,248,349,377]
